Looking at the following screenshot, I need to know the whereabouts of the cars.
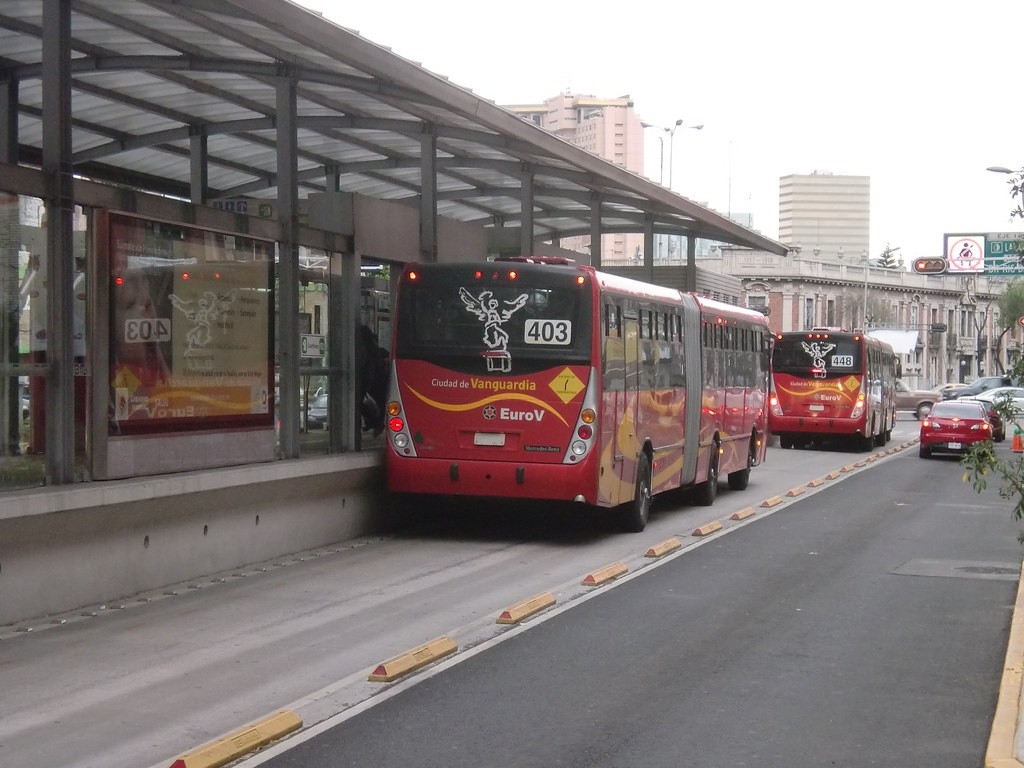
[974,387,1024,416]
[896,379,940,420]
[959,394,1006,443]
[942,376,1020,400]
[919,398,994,461]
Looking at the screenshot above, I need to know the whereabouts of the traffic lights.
[910,255,948,275]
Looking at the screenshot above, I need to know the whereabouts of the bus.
[376,251,781,536]
[769,325,900,449]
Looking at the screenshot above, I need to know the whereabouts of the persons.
[360,325,390,436]
[142,305,172,407]
